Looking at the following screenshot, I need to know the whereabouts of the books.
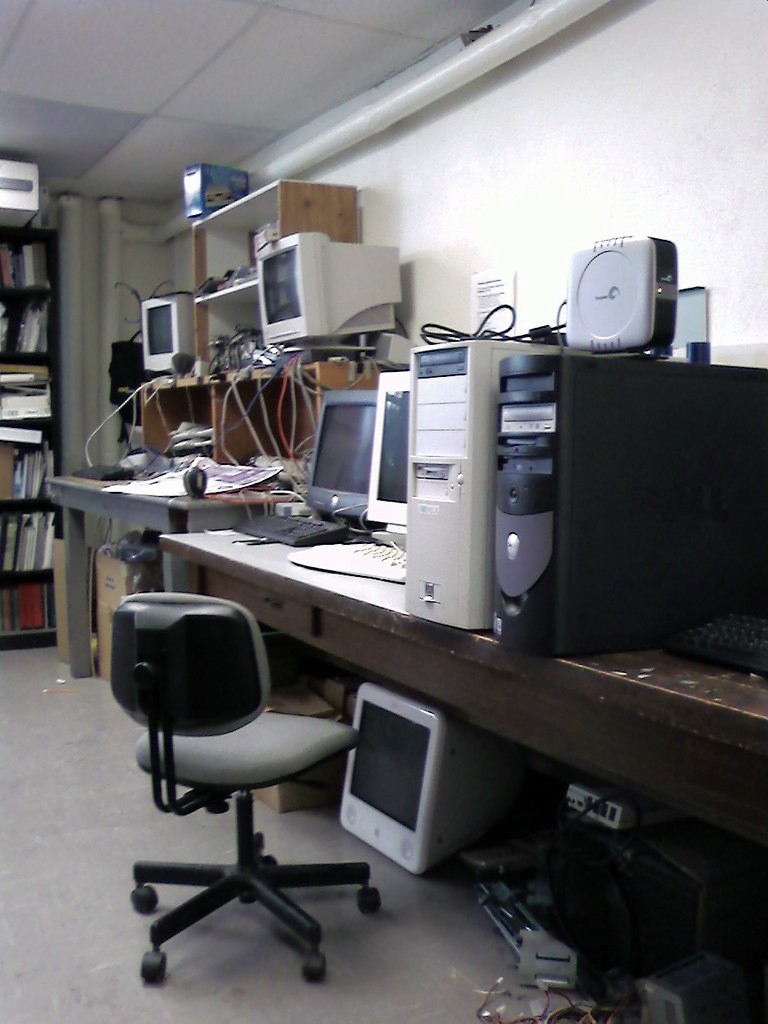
[0,242,56,633]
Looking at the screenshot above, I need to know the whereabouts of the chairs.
[108,591,381,988]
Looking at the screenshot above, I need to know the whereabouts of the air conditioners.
[0,160,40,227]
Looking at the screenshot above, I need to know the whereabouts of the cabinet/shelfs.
[193,179,359,374]
[0,227,62,651]
[140,359,379,465]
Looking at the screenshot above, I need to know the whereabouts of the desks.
[44,476,297,678]
[157,529,766,847]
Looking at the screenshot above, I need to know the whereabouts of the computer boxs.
[409,341,768,660]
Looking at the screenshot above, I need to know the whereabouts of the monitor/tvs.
[142,293,197,379]
[257,232,403,351]
[340,682,521,875]
[307,390,379,540]
[367,371,412,546]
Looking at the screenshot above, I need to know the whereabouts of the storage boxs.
[183,162,251,221]
[251,677,358,814]
[94,551,150,680]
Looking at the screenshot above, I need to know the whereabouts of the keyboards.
[233,514,348,547]
[72,465,134,482]
[664,611,768,678]
[287,543,407,582]
